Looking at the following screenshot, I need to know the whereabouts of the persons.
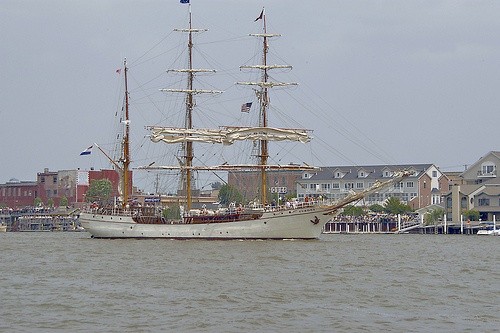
[3,194,412,231]
[466,218,470,224]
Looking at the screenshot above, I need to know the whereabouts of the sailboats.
[79,0,416,238]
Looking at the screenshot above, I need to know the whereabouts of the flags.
[241,101,253,112]
[80,145,93,155]
[179,1,191,4]
[252,9,264,23]
